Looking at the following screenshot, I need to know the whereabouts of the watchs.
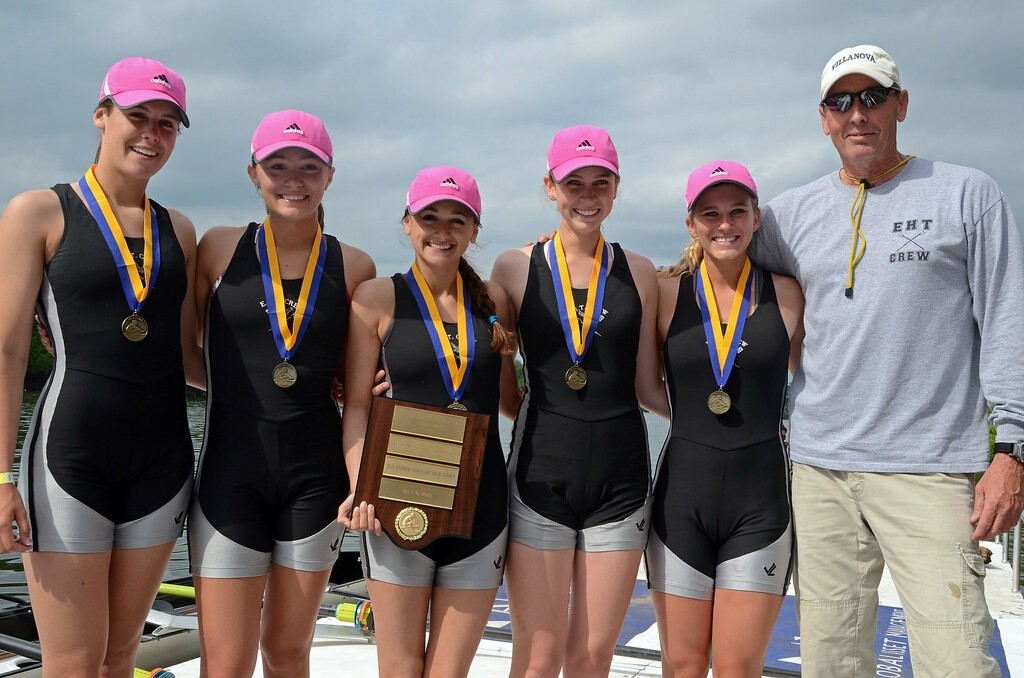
[994,441,1024,464]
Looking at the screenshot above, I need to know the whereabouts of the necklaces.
[843,156,917,297]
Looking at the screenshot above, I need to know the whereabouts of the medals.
[707,388,731,414]
[565,361,587,390]
[121,312,148,341]
[447,401,468,411]
[272,360,297,388]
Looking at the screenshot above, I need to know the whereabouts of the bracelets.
[0,472,15,485]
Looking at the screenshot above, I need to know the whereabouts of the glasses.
[819,84,903,112]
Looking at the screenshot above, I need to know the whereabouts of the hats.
[97,57,190,129]
[403,166,482,222]
[820,44,903,106]
[684,160,759,213]
[250,109,333,167]
[547,124,618,184]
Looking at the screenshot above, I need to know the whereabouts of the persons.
[371,126,672,678]
[337,168,520,678]
[643,160,807,678]
[0,56,207,678]
[527,44,1024,678]
[36,109,375,678]
